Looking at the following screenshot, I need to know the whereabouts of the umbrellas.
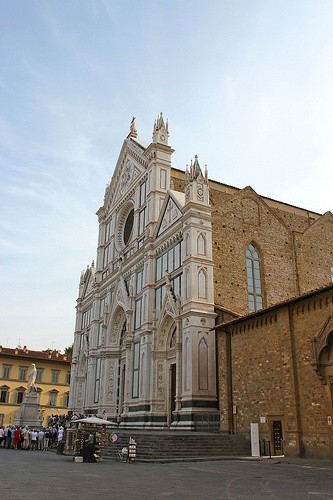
[71,415,118,446]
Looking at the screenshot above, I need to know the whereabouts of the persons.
[49,409,109,427]
[0,424,65,450]
[26,363,38,391]
[121,445,129,463]
[83,429,96,464]
